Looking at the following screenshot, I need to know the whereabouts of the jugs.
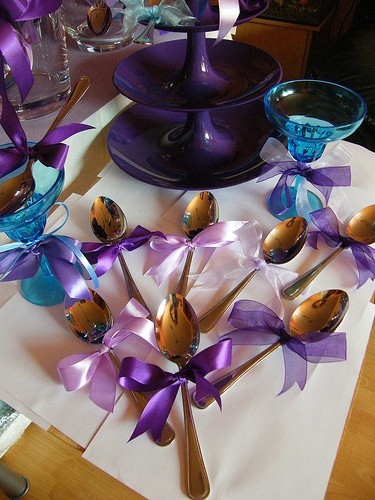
[58,0,158,53]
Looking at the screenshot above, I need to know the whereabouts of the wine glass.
[263,80,366,223]
[0,141,83,306]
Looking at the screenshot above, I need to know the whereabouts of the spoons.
[281,204,375,300]
[63,287,176,446]
[174,191,220,296]
[192,289,349,408]
[132,0,174,47]
[87,1,112,37]
[89,196,152,320]
[0,76,92,218]
[197,216,309,334]
[155,292,210,500]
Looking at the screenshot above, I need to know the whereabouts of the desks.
[233,5,336,104]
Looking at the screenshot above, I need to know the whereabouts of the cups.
[0,10,72,122]
